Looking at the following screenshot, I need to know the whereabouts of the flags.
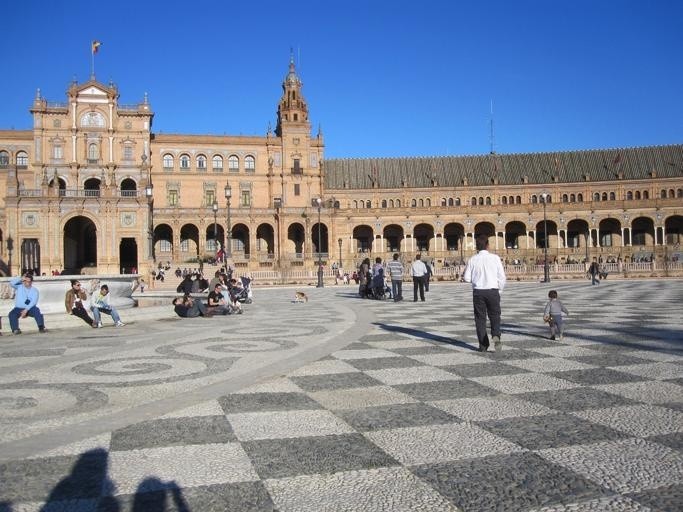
[91,40,104,55]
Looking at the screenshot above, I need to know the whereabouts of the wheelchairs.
[365,274,391,299]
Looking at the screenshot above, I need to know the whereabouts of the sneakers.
[114,320,126,328]
[476,344,488,352]
[12,326,47,334]
[360,289,430,302]
[492,334,503,353]
[97,321,104,328]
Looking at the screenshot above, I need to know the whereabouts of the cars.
[604,251,682,263]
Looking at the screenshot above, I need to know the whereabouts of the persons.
[358,257,370,278]
[156,260,242,321]
[588,257,599,284]
[64,279,98,327]
[422,261,433,291]
[542,290,568,340]
[139,279,144,292]
[409,253,427,301]
[386,253,404,301]
[598,267,607,279]
[504,252,668,264]
[89,285,125,329]
[7,274,46,333]
[320,260,359,286]
[461,234,506,353]
[371,257,386,279]
[372,267,393,300]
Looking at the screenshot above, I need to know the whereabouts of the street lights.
[316,198,324,287]
[458,232,464,265]
[337,237,343,268]
[223,177,233,258]
[211,197,220,263]
[540,191,550,283]
[143,182,156,261]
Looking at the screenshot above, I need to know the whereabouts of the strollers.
[231,275,253,305]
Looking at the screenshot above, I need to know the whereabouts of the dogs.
[296,292,307,304]
